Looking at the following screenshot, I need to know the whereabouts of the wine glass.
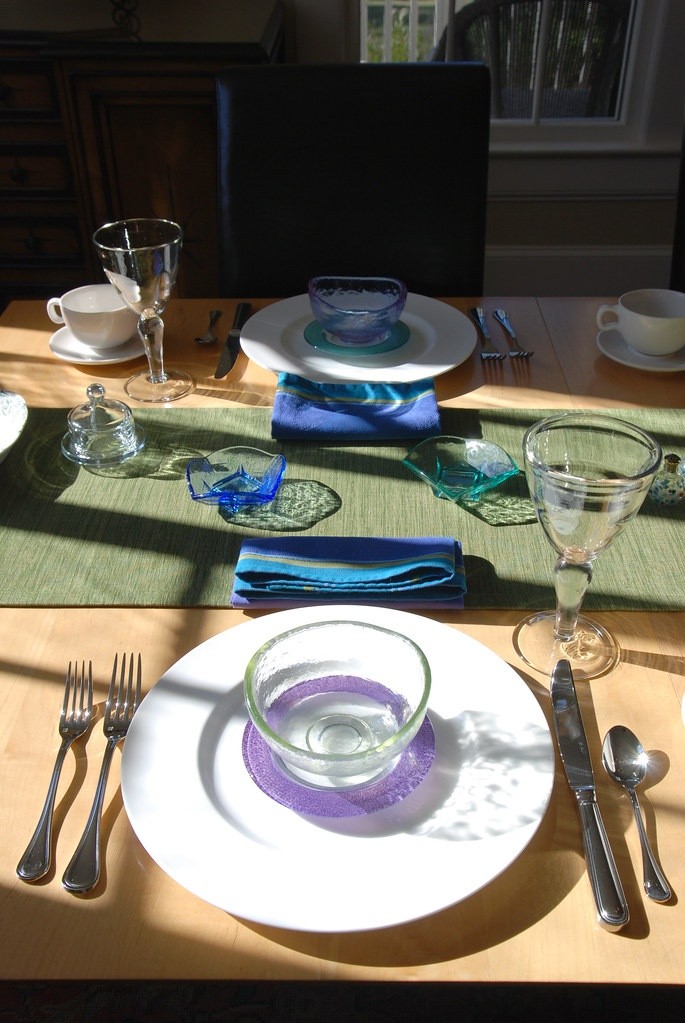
[514,414,662,681]
[91,217,191,403]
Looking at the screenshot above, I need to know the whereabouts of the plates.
[241,292,478,385]
[49,327,145,365]
[597,328,685,372]
[123,603,558,934]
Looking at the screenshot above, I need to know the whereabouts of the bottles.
[648,455,685,506]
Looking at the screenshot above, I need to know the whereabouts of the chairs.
[213,60,492,297]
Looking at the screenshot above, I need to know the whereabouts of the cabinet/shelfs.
[0,28,274,309]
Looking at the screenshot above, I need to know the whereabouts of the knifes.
[212,303,252,378]
[549,660,630,933]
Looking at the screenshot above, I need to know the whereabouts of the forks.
[467,306,506,359]
[15,659,93,881]
[63,652,140,893]
[492,310,534,357]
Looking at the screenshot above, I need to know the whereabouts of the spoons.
[193,309,223,344]
[601,726,672,902]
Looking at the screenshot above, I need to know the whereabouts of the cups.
[597,289,685,355]
[46,283,141,350]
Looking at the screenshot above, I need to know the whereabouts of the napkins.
[270,370,442,443]
[230,536,468,611]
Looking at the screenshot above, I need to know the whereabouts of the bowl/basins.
[241,620,431,786]
[307,277,408,343]
[0,388,29,463]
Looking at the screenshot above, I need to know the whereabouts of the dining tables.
[0,295,685,1023]
